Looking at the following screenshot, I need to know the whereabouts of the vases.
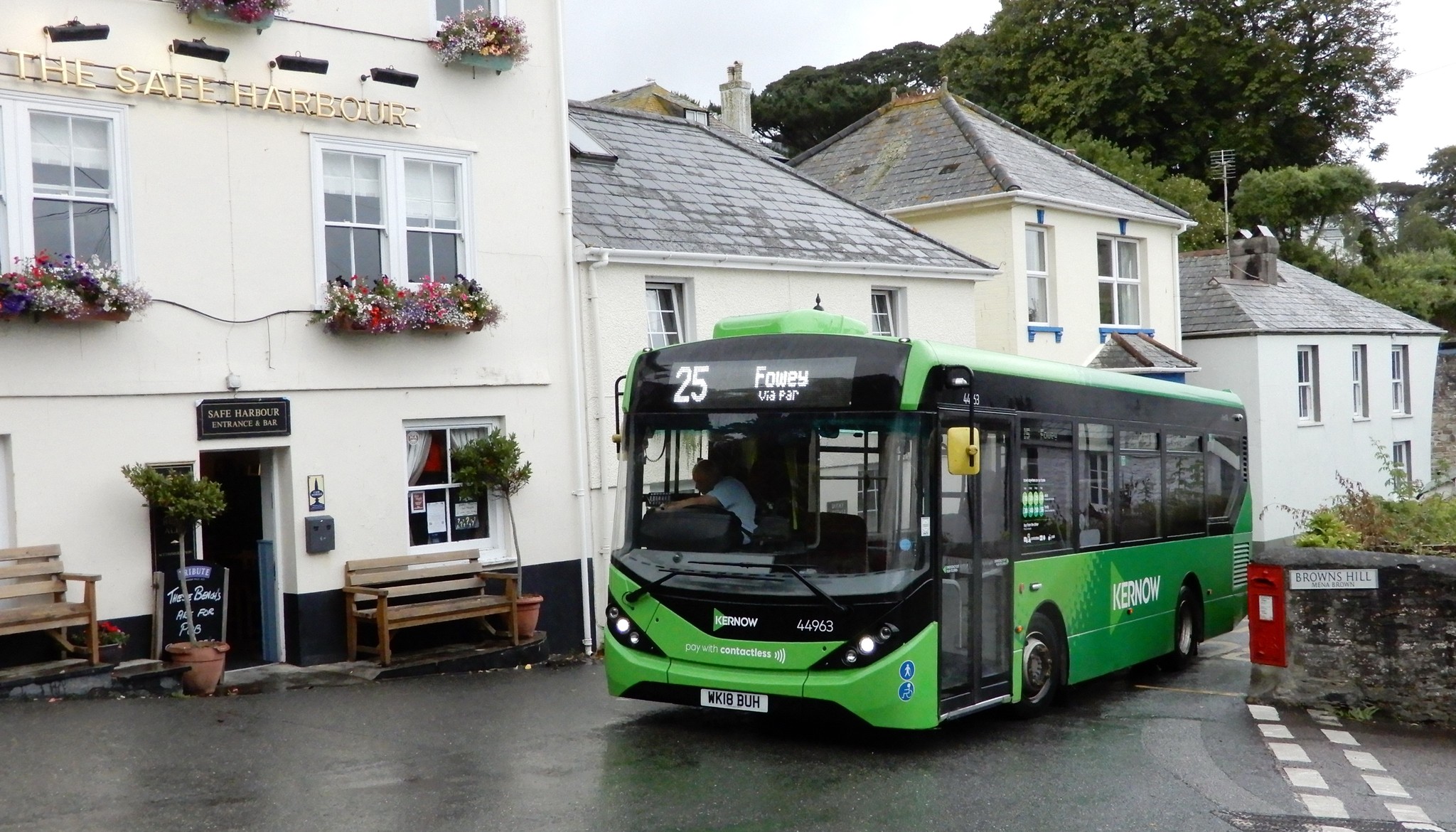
[98,644,120,666]
[200,6,274,29]
[461,51,516,71]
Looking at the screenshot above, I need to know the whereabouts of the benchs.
[0,545,101,666]
[343,547,520,665]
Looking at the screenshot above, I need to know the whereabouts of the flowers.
[72,621,130,645]
[436,11,527,57]
[0,253,151,320]
[178,0,289,15]
[323,276,495,333]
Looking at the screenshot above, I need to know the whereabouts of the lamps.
[269,55,329,74]
[361,68,419,87]
[169,38,230,62]
[43,21,109,43]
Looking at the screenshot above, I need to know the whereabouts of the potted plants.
[121,462,229,697]
[450,431,544,639]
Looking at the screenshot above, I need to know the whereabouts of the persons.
[658,459,758,548]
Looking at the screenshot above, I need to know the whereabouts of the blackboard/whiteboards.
[152,559,229,653]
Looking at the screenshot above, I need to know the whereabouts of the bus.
[604,311,1253,730]
